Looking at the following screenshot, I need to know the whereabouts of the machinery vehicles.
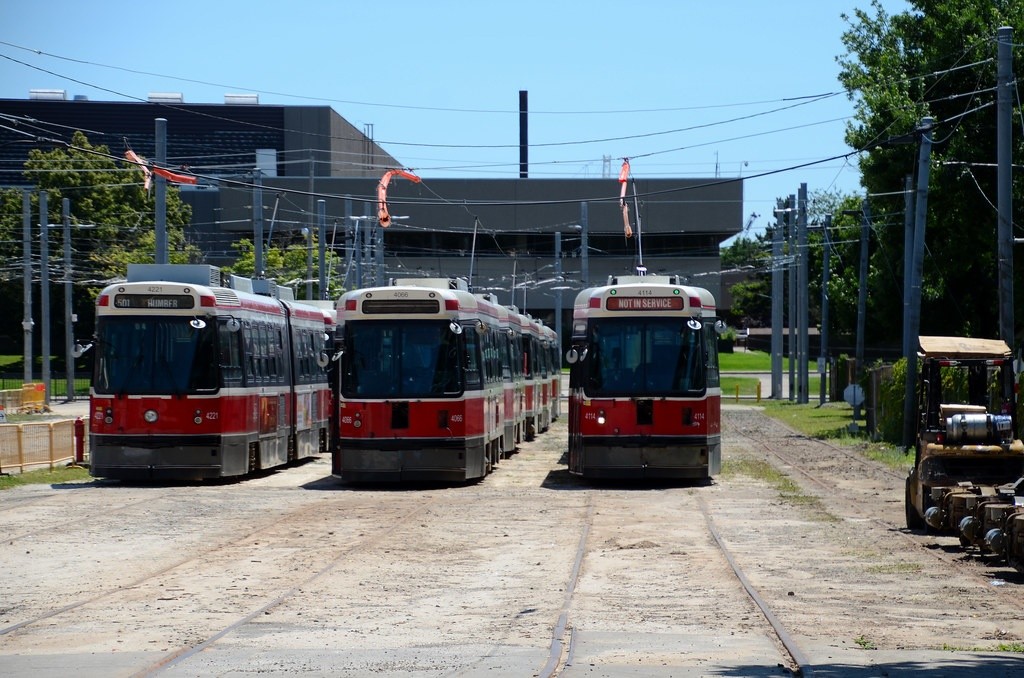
[903,335,1023,572]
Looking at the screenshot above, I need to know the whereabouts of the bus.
[86,261,338,485]
[315,275,563,490]
[567,265,722,484]
